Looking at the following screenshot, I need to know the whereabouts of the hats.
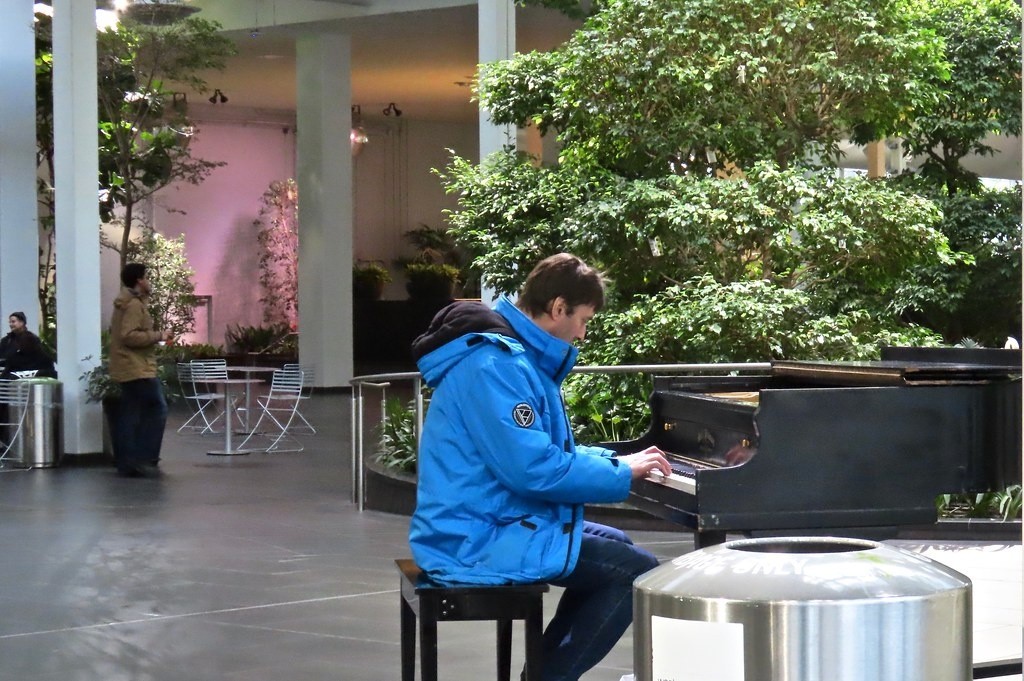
[9,312,26,324]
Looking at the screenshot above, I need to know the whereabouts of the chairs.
[174,355,316,458]
[0,379,35,472]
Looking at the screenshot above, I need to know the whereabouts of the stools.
[393,556,547,680]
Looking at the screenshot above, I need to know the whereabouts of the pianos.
[584,344,1022,552]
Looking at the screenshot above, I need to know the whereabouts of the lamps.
[209,89,229,106]
[383,102,402,116]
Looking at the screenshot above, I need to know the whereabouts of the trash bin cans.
[8,376,66,469]
[631,533,976,679]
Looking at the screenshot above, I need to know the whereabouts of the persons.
[413,251,671,681]
[112,262,175,477]
[0,310,57,378]
